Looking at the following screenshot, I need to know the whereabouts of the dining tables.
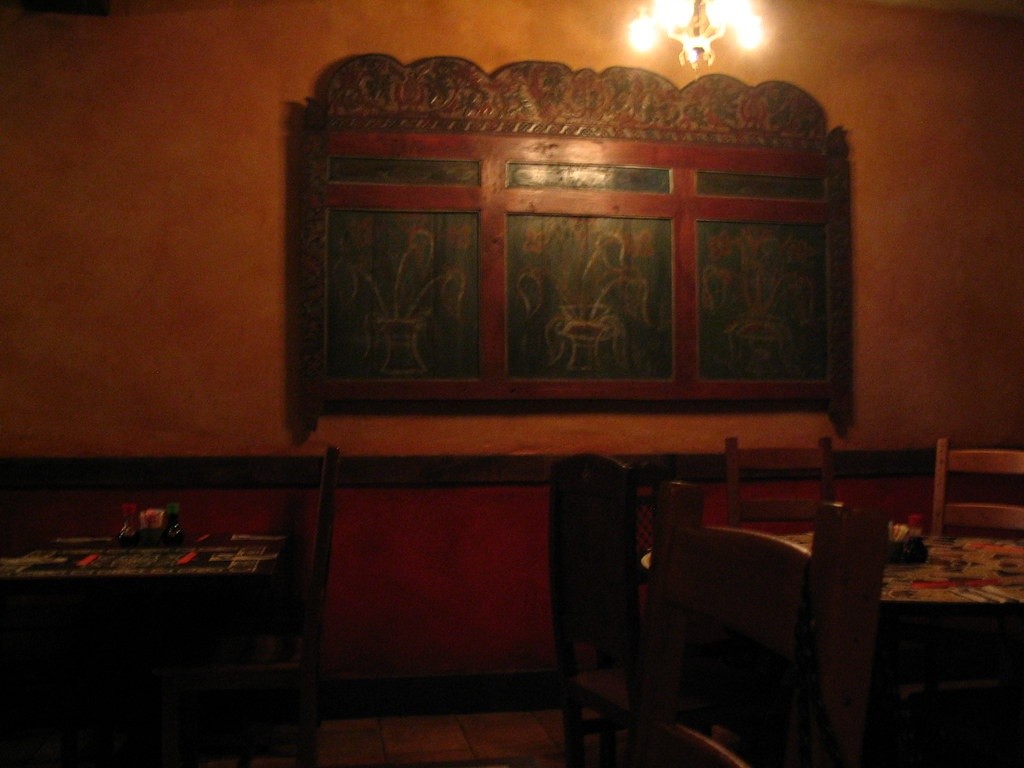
[0,528,289,768]
[771,530,1024,768]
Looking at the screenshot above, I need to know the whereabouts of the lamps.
[627,0,766,70]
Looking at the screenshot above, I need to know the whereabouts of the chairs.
[547,433,1024,768]
[146,445,341,767]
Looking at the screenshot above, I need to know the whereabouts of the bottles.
[903,514,927,562]
[162,503,185,547]
[116,502,139,547]
